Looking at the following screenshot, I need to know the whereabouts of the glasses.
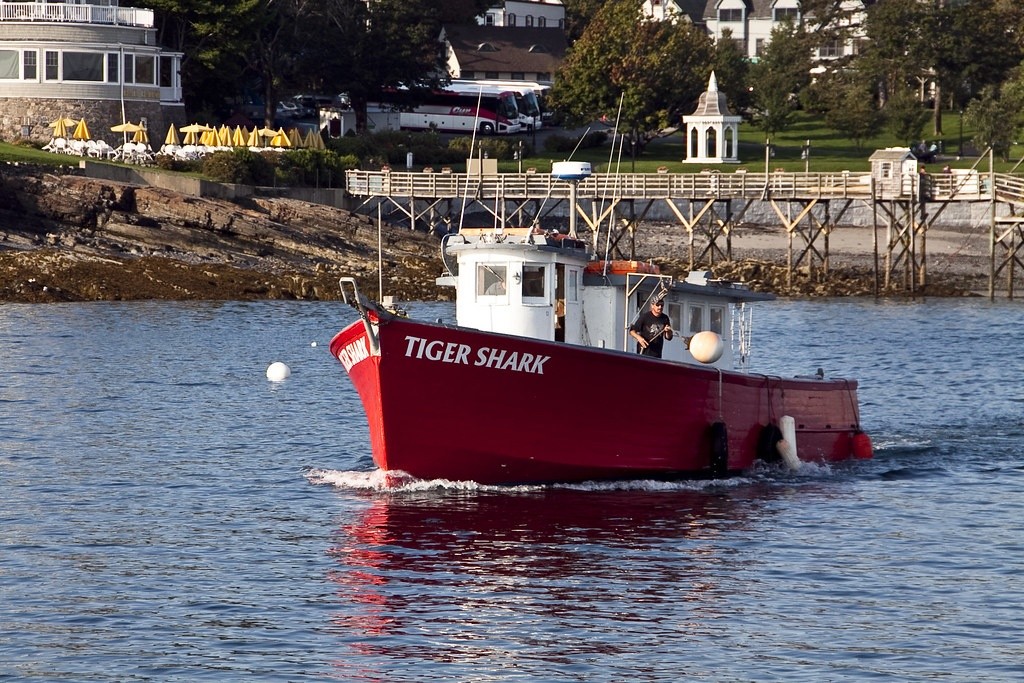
[655,304,663,307]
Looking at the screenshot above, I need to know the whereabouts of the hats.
[652,297,664,306]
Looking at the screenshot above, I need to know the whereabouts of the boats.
[328,85,874,482]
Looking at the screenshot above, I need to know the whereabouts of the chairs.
[42,138,293,164]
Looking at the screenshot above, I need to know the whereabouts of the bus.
[399,76,564,135]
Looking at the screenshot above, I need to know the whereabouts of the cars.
[274,93,333,123]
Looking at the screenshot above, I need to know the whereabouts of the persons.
[920,166,927,176]
[920,140,927,152]
[930,141,937,157]
[943,165,951,173]
[483,270,506,295]
[629,299,673,358]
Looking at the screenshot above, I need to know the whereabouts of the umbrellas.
[183,124,196,145]
[270,127,291,148]
[165,123,179,145]
[49,118,78,128]
[53,118,67,137]
[302,128,326,150]
[111,122,146,143]
[288,127,303,149]
[200,123,260,147]
[133,121,148,143]
[73,118,91,139]
[249,125,277,147]
[179,125,213,132]
